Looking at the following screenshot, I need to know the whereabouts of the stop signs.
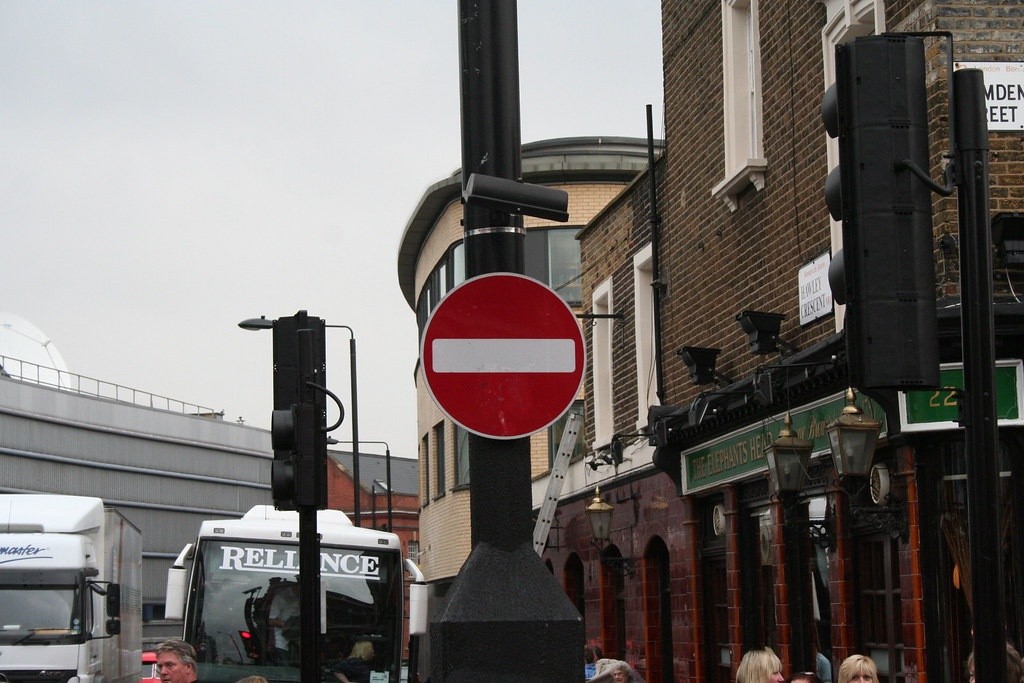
[420,272,588,441]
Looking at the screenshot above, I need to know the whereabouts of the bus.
[164,506,429,683]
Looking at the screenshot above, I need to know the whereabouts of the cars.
[142,653,164,683]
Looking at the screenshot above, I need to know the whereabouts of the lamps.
[584,486,637,579]
[678,346,734,388]
[824,386,910,543]
[734,310,799,360]
[762,410,837,553]
[689,390,752,427]
[752,360,832,407]
[993,211,1024,270]
[650,414,689,449]
[588,431,650,472]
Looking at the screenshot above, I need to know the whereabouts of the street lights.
[238,315,361,528]
[217,631,243,665]
[328,436,391,533]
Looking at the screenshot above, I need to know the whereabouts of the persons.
[968,642,1024,683]
[736,647,784,683]
[584,645,634,683]
[838,654,879,683]
[331,639,376,683]
[154,639,200,683]
[789,673,822,683]
[817,651,831,683]
[236,676,267,683]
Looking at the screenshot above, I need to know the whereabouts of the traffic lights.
[270,404,328,511]
[238,631,257,658]
[820,34,938,388]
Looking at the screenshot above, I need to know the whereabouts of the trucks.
[0,493,142,683]
